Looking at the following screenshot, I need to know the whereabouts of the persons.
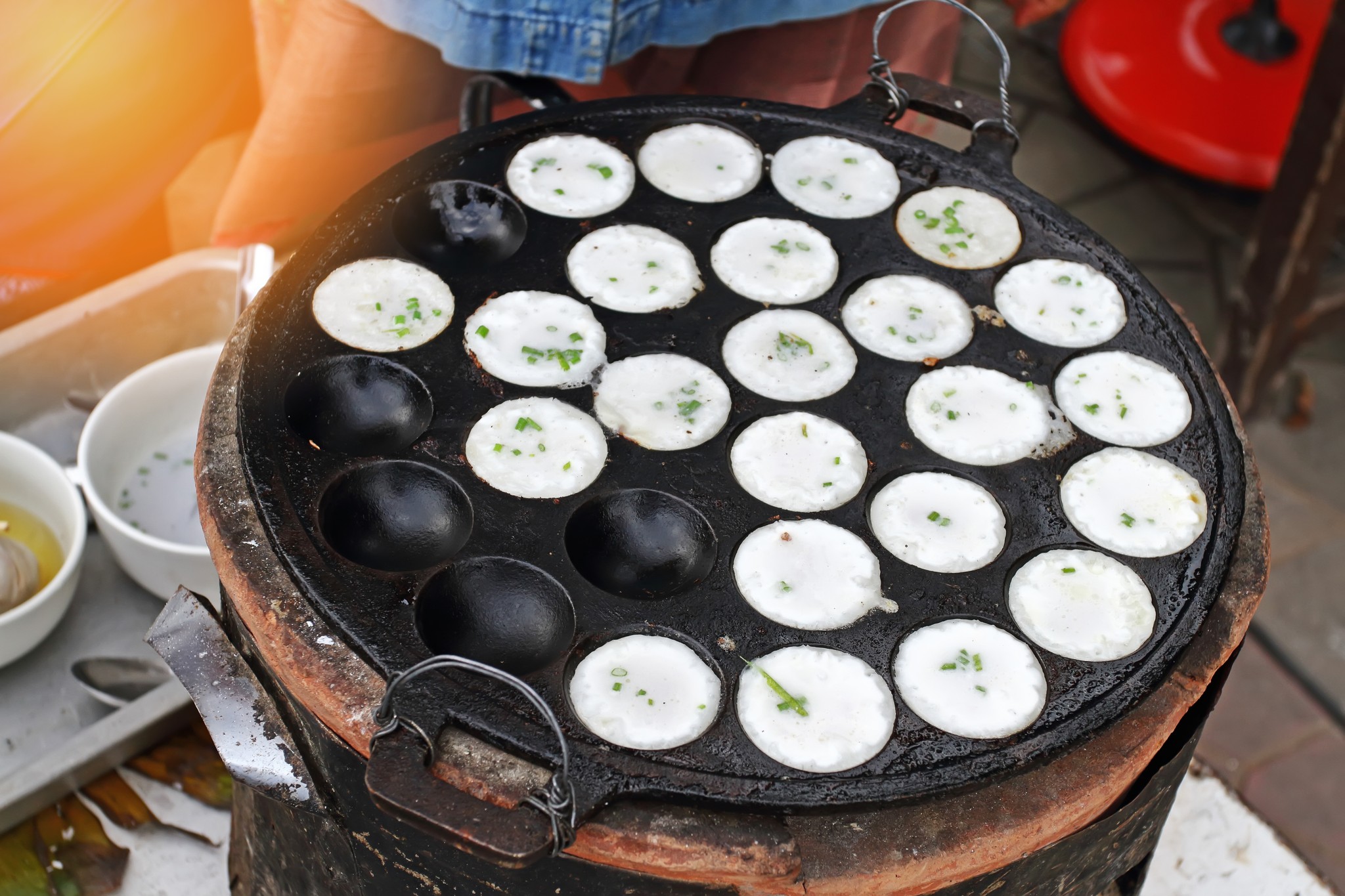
[211,2,965,257]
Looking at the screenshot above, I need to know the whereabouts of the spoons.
[69,658,172,707]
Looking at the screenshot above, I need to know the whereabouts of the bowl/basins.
[77,344,223,618]
[1,427,88,676]
